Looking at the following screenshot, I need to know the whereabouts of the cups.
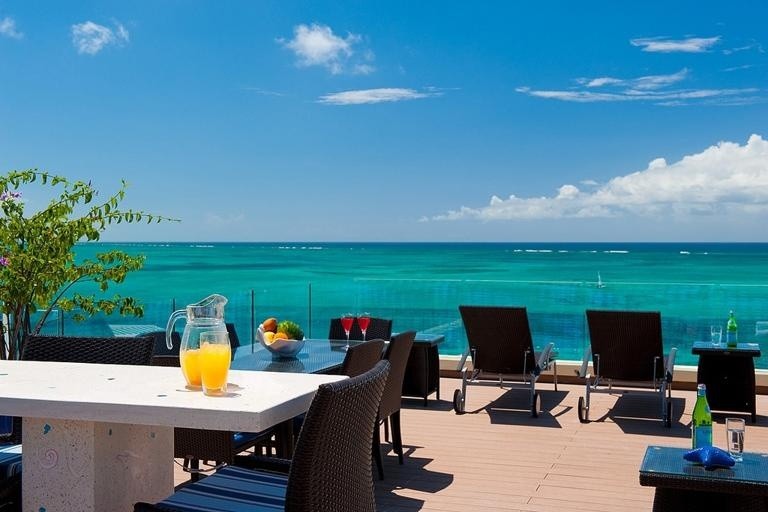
[711,325,722,347]
[199,331,231,397]
[725,418,745,463]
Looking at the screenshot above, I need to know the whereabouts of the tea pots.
[165,294,228,391]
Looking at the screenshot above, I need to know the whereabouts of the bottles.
[726,312,738,347]
[691,383,713,449]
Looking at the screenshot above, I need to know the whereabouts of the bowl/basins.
[255,327,304,358]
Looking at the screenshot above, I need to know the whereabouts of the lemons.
[273,332,287,341]
[264,332,274,341]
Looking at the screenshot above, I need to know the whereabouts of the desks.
[229,340,388,458]
[639,445,767,512]
[693,340,761,424]
[393,331,445,409]
[0,359,350,510]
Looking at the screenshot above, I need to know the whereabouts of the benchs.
[0,334,159,511]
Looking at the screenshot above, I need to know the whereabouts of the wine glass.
[338,311,356,350]
[357,311,371,342]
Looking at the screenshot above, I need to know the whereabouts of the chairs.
[285,339,386,480]
[577,308,678,428]
[372,331,416,467]
[327,315,393,341]
[173,422,277,485]
[135,330,181,366]
[451,304,561,418]
[131,359,391,511]
[225,323,240,349]
